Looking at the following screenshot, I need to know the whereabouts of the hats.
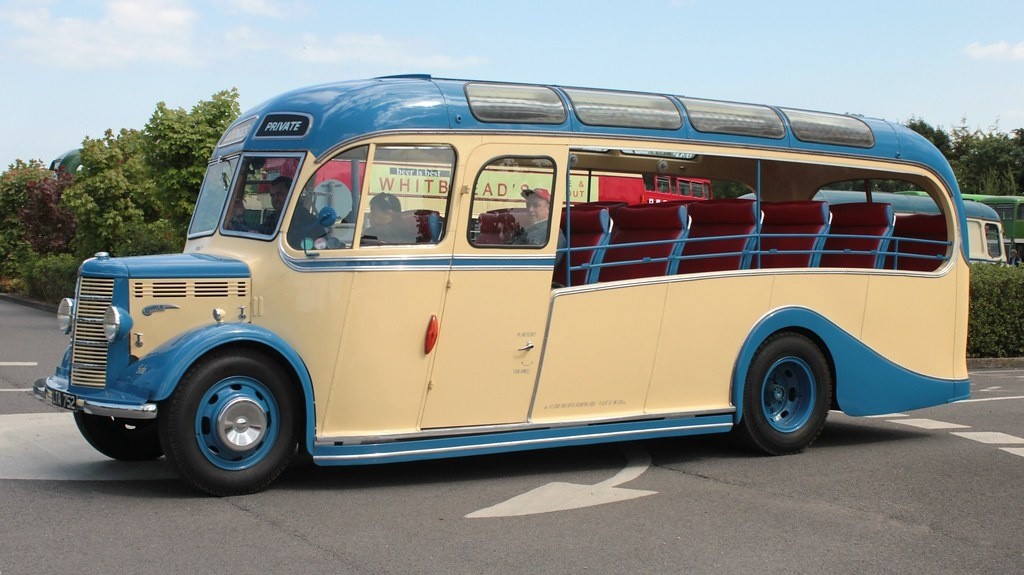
[520,188,551,204]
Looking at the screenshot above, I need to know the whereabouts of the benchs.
[561,205,692,283]
[884,213,947,271]
[750,200,833,269]
[362,209,441,245]
[473,206,615,285]
[630,199,765,274]
[820,202,897,269]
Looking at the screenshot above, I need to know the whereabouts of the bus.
[22,72,978,498]
[894,190,1024,270]
[730,188,1011,270]
[253,152,715,271]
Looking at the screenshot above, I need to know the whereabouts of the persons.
[357,191,425,246]
[1009,249,1021,267]
[510,187,568,266]
[246,176,327,251]
